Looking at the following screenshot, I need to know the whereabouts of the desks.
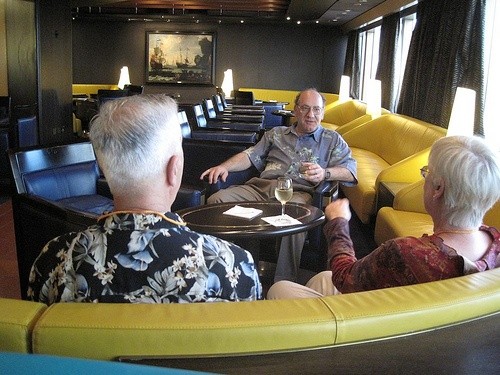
[174,201,326,265]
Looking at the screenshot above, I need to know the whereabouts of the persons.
[266,136,500,300]
[26,95,264,304]
[200,89,358,284]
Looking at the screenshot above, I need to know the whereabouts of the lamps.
[117,66,132,90]
[221,69,234,99]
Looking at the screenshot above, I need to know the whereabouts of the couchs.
[342,112,447,227]
[322,95,391,136]
[238,88,352,125]
[2,173,499,375]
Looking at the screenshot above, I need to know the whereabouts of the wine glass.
[275,177,293,222]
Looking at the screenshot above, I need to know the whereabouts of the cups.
[299,160,313,178]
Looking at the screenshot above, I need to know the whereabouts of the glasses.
[297,103,322,112]
[420,166,429,177]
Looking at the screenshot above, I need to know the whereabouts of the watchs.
[324,168,330,181]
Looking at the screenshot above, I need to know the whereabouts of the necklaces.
[436,230,474,235]
[97,209,187,226]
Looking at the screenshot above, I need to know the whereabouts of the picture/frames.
[144,30,217,87]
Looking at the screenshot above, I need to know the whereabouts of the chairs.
[0,83,264,301]
